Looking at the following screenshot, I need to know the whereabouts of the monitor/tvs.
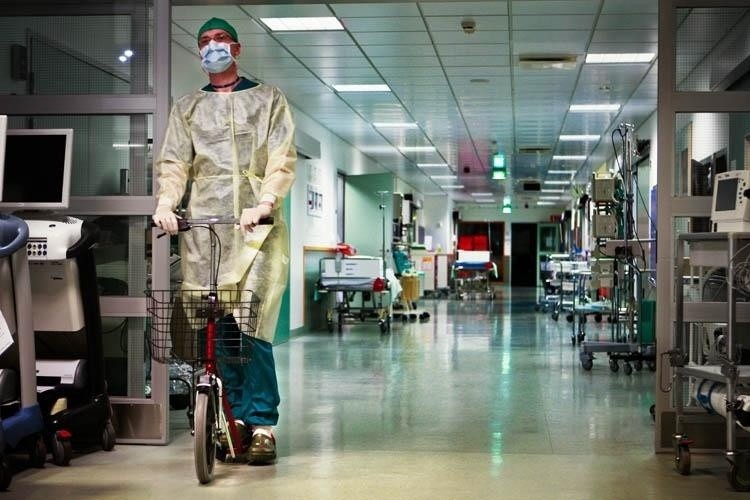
[0,128,76,210]
[710,170,749,233]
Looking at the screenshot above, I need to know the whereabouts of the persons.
[151,16,298,465]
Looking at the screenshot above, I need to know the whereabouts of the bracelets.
[255,201,274,208]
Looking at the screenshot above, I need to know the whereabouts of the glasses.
[198,33,234,45]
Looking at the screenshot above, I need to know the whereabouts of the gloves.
[152,206,182,236]
[235,205,272,232]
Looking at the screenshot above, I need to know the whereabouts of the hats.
[198,17,238,42]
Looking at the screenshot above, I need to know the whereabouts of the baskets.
[146,290,260,365]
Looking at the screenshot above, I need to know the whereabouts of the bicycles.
[148,206,278,490]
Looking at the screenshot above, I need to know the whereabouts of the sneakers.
[246,432,276,463]
[219,423,251,449]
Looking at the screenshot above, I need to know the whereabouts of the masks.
[199,40,239,74]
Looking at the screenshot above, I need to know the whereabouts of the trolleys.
[0,210,52,497]
[388,215,431,323]
[665,231,748,492]
[7,213,121,469]
[535,121,656,377]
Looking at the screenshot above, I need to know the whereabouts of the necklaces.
[209,77,240,88]
